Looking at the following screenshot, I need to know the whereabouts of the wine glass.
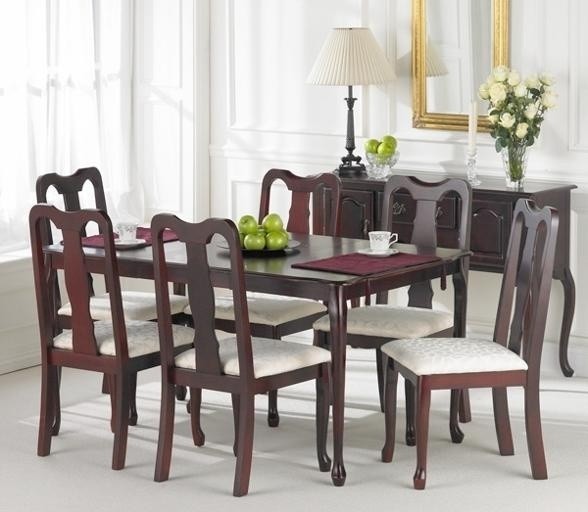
[114,222,138,239]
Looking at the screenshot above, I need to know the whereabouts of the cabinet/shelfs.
[315,175,577,378]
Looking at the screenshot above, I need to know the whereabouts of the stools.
[311,174,472,447]
[152,213,331,497]
[184,168,342,427]
[381,198,559,489]
[30,204,204,469]
[36,167,189,426]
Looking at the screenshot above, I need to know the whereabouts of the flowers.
[478,69,555,180]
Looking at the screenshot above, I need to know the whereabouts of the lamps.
[304,26,399,173]
[426,33,451,78]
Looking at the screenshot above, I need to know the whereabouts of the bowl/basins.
[365,164,390,178]
[366,150,399,169]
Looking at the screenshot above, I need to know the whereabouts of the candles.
[468,100,477,155]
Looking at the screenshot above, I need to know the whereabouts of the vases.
[495,139,527,191]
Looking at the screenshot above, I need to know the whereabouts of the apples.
[377,142,393,155]
[238,214,289,251]
[365,139,379,152]
[382,136,398,147]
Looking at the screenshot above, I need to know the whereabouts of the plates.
[355,248,399,259]
[112,238,147,250]
[215,239,301,252]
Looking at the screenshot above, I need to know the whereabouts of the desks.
[41,225,474,486]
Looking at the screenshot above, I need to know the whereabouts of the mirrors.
[410,1,511,134]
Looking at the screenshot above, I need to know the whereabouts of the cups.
[369,230,399,252]
